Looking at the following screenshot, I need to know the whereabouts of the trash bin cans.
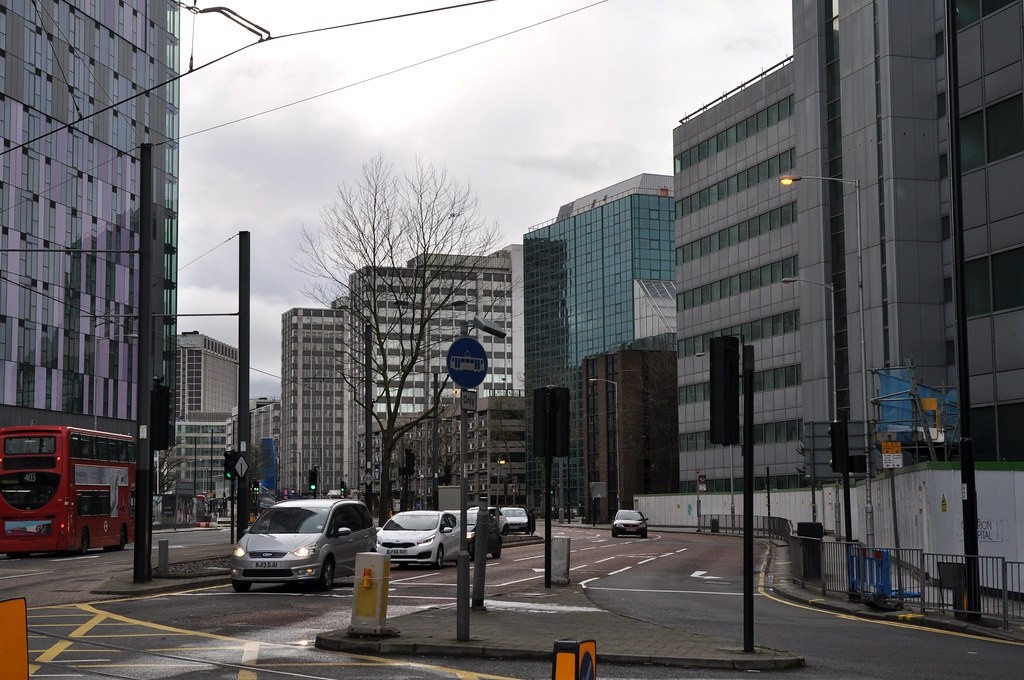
[797,522,824,581]
[710,518,720,532]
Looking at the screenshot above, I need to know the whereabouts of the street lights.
[781,276,842,543]
[779,173,876,559]
[697,352,735,531]
[457,315,507,640]
[497,459,505,507]
[589,379,620,514]
[94,334,139,429]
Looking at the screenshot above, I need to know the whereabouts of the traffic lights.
[151,384,173,451]
[341,481,344,493]
[407,451,415,476]
[254,481,258,492]
[828,422,842,474]
[309,470,317,491]
[224,451,236,480]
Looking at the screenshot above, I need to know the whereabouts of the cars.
[499,507,530,535]
[375,511,461,571]
[442,509,503,562]
[468,506,509,536]
[230,501,379,593]
[612,510,648,539]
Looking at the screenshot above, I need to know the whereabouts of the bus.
[0,426,134,558]
